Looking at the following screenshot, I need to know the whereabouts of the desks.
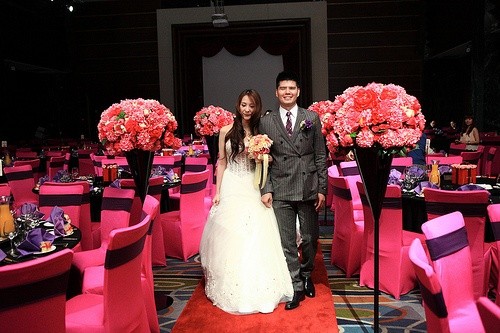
[121,172,179,187]
[390,173,499,231]
[31,179,111,220]
[0,220,82,267]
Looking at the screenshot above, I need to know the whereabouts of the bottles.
[0,196,15,237]
[188,144,193,155]
[5,153,11,165]
[428,159,440,187]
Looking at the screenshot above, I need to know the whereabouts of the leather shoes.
[285,291,305,310]
[299,273,315,297]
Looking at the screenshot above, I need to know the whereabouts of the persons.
[455,112,480,164]
[425,120,441,130]
[258,73,328,310]
[199,89,294,316]
[447,119,462,135]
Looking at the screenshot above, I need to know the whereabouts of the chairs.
[323,130,500,333]
[0,136,216,333]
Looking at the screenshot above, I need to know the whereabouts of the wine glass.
[3,220,18,254]
[72,167,78,178]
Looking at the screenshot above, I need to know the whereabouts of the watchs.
[468,141,471,144]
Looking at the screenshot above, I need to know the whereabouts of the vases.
[206,133,231,165]
[124,148,157,203]
[352,142,395,332]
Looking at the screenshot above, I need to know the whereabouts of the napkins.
[438,165,452,172]
[112,179,121,187]
[37,174,52,185]
[165,169,176,183]
[458,184,484,192]
[415,181,440,194]
[19,202,44,220]
[55,169,69,181]
[176,149,185,155]
[196,148,201,153]
[16,228,55,256]
[386,174,400,183]
[152,166,164,175]
[407,164,426,176]
[54,222,71,237]
[1,250,6,261]
[45,207,67,224]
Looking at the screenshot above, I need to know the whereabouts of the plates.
[33,245,56,254]
[43,221,74,237]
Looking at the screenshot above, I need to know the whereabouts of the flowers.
[97,83,422,163]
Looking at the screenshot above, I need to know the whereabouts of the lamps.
[210,0,229,26]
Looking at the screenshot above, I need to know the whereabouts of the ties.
[286,112,293,137]
